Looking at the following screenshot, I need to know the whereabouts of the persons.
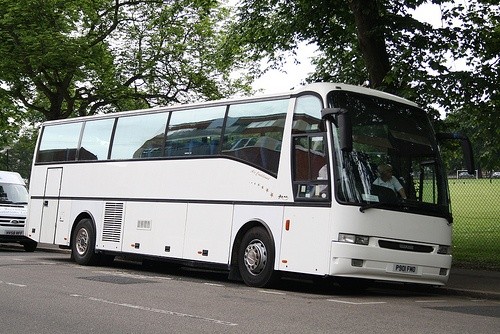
[372,164,407,202]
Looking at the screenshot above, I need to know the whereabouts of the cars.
[459,172,474,179]
[492,172,500,179]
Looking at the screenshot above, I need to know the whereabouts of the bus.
[24,83,476,289]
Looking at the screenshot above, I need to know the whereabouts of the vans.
[0,171,38,252]
